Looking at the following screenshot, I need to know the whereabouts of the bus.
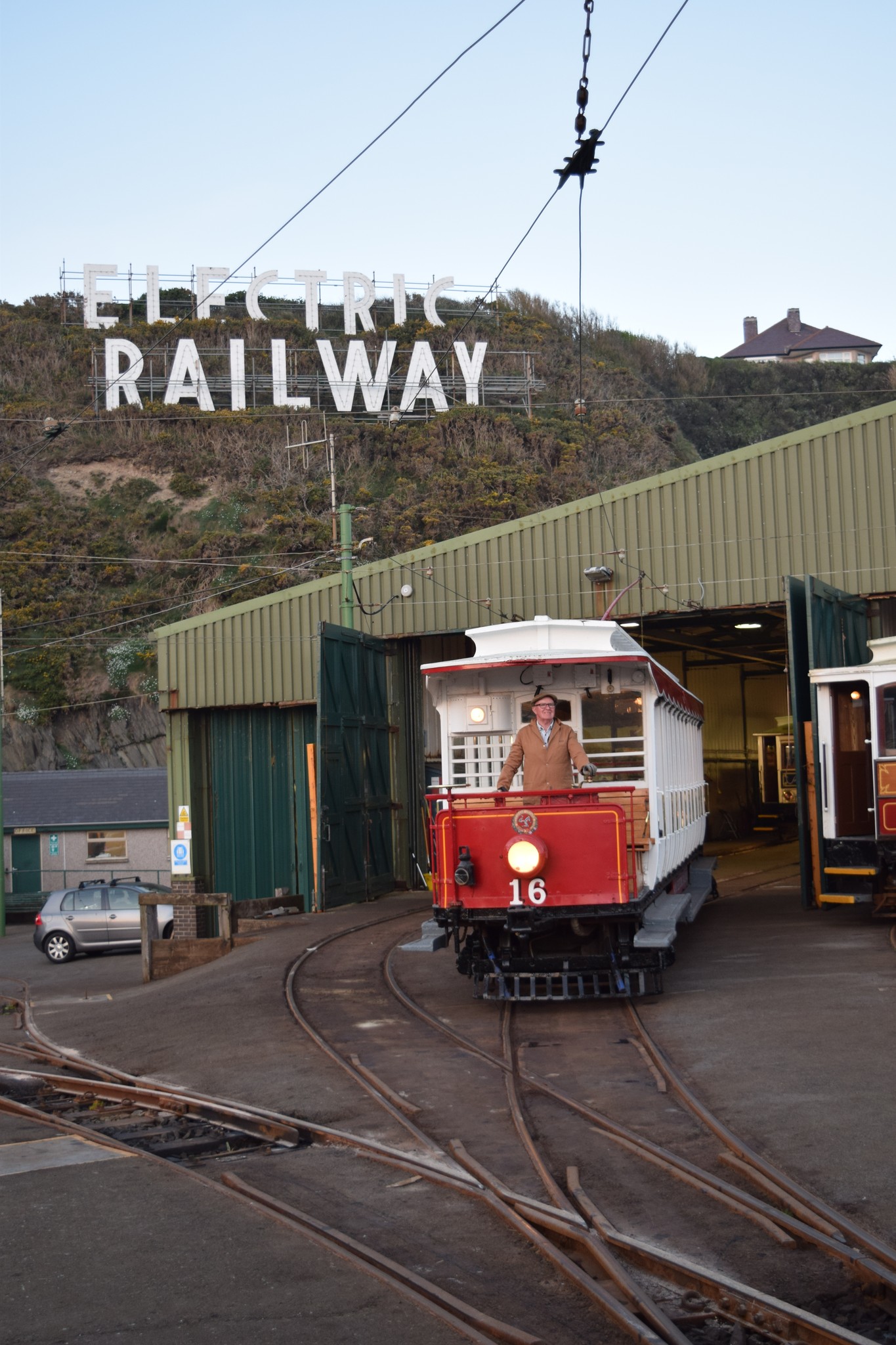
[416,614,717,1009]
[808,631,896,927]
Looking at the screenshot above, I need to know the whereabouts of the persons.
[497,692,597,806]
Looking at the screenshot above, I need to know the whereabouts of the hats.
[530,692,558,708]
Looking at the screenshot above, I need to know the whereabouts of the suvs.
[31,875,175,965]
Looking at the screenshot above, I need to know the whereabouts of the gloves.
[582,763,597,776]
[491,789,503,800]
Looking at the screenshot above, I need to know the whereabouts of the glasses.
[533,703,556,708]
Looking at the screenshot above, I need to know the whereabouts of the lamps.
[583,566,612,582]
